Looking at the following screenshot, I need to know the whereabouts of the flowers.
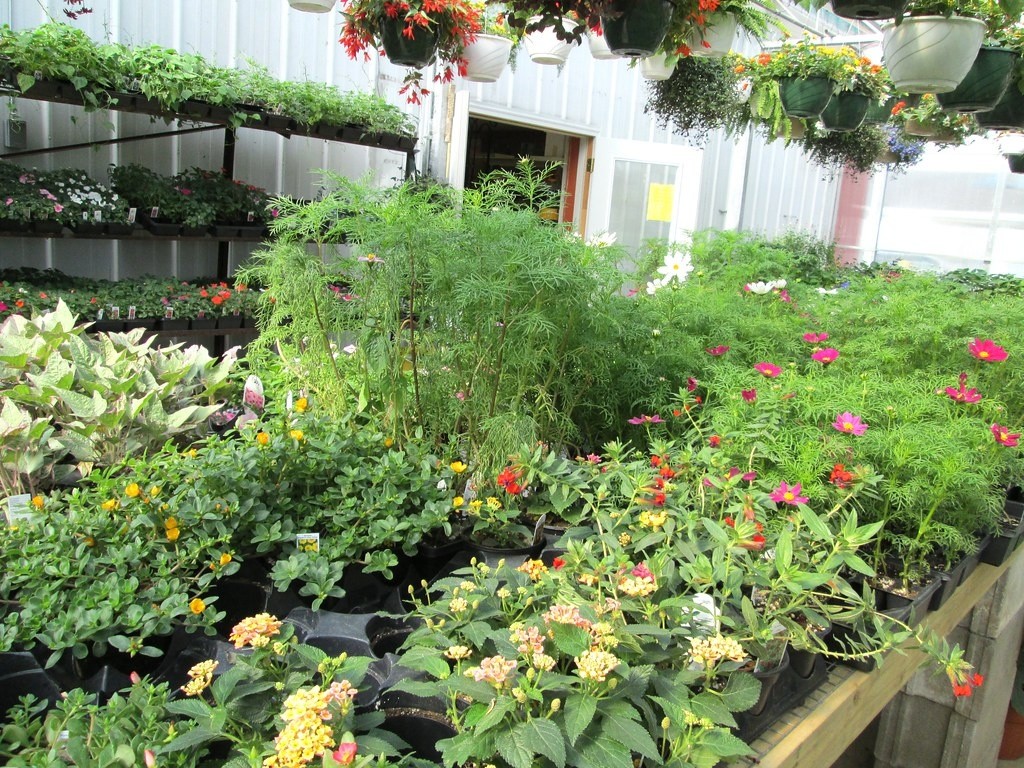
[466,0,528,72]
[726,0,1024,173]
[0,164,280,229]
[0,266,277,321]
[508,0,722,68]
[340,0,483,106]
[2,330,1019,765]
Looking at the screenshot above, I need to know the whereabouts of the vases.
[821,90,872,135]
[96,319,265,331]
[460,33,515,83]
[936,45,1022,116]
[377,15,446,70]
[832,0,911,21]
[975,60,1024,134]
[641,47,680,80]
[1008,153,1024,173]
[1,496,1022,768]
[524,16,583,66]
[586,17,620,60]
[874,144,902,164]
[860,90,896,124]
[878,15,989,95]
[932,125,958,145]
[0,219,277,236]
[777,74,837,118]
[602,1,675,59]
[905,117,940,138]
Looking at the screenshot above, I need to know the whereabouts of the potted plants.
[690,0,788,59]
[0,22,420,153]
